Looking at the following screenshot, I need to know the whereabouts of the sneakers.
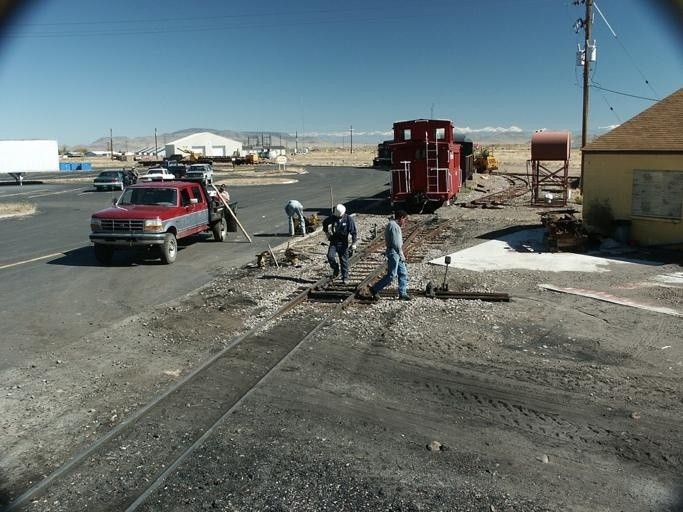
[400,295,414,301]
[369,287,380,300]
[333,263,339,278]
[343,277,348,281]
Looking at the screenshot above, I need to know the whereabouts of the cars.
[89,147,215,192]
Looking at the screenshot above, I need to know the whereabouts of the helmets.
[335,203,346,217]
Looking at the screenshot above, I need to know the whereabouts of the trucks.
[229,150,271,166]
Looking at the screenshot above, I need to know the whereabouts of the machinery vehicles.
[472,145,499,174]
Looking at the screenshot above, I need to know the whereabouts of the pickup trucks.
[87,179,238,264]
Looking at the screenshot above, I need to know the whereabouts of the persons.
[321,203,356,284]
[213,184,230,204]
[284,199,306,237]
[364,208,413,301]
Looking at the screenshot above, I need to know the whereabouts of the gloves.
[400,253,406,262]
[349,243,357,251]
[325,231,330,238]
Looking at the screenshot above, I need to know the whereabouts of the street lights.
[571,17,592,196]
[152,126,159,156]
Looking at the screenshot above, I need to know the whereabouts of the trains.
[376,115,473,204]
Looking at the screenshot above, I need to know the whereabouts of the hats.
[220,184,225,190]
